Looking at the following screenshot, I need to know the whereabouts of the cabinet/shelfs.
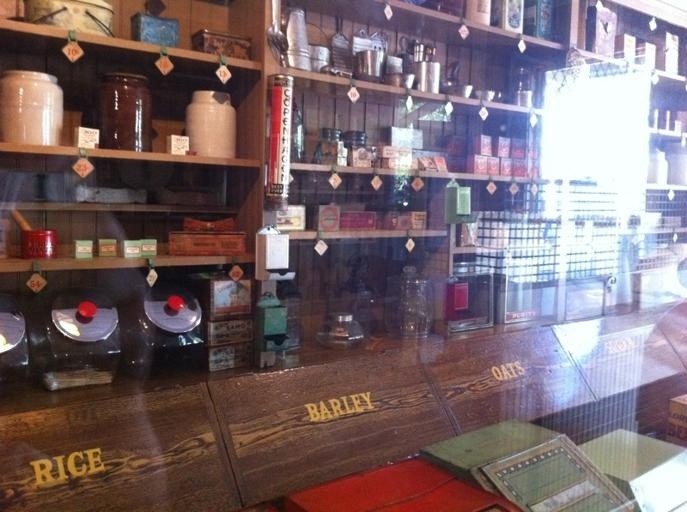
[569,1,685,270]
[272,1,573,301]
[1,0,275,348]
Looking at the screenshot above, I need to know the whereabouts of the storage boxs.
[190,28,254,64]
[584,2,619,60]
[162,231,251,259]
[126,11,181,51]
[613,30,636,69]
[308,202,431,233]
[187,270,252,321]
[372,125,542,184]
[200,337,256,373]
[654,28,681,78]
[197,315,255,347]
[634,38,657,72]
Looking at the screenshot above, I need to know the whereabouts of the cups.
[384,73,402,87]
[357,49,383,82]
[94,71,154,153]
[464,1,492,27]
[414,62,440,94]
[514,90,531,108]
[0,69,65,147]
[21,229,56,259]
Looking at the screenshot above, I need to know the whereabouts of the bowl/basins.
[474,91,494,100]
[456,85,472,97]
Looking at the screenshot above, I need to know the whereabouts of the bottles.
[281,8,311,71]
[413,40,436,63]
[182,88,238,158]
[652,151,669,186]
[344,130,371,168]
[312,128,347,166]
[382,265,434,340]
[313,312,364,349]
[278,270,304,352]
[398,36,419,90]
[0,284,204,397]
[290,95,304,162]
